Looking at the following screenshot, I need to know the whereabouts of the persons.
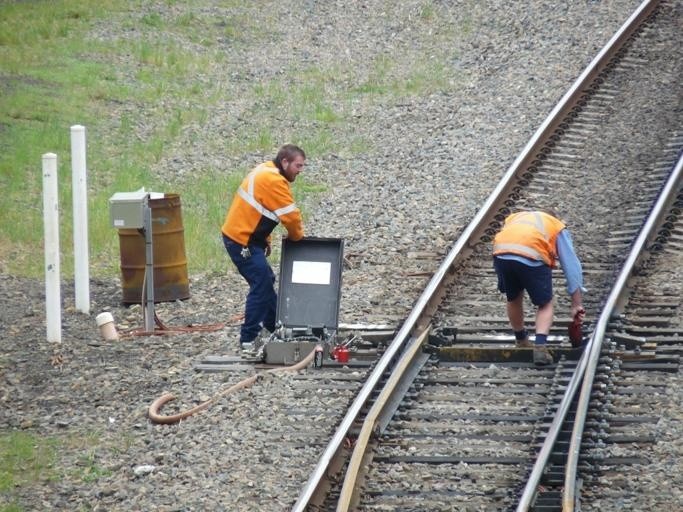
[219,142,306,353]
[489,209,585,367]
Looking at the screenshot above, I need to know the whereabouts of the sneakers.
[514,335,533,350]
[531,344,555,365]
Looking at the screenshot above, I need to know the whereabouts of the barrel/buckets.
[118,193,193,304]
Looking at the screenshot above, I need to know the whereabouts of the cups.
[338,349,349,363]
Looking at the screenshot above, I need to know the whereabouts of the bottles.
[314,344,323,369]
